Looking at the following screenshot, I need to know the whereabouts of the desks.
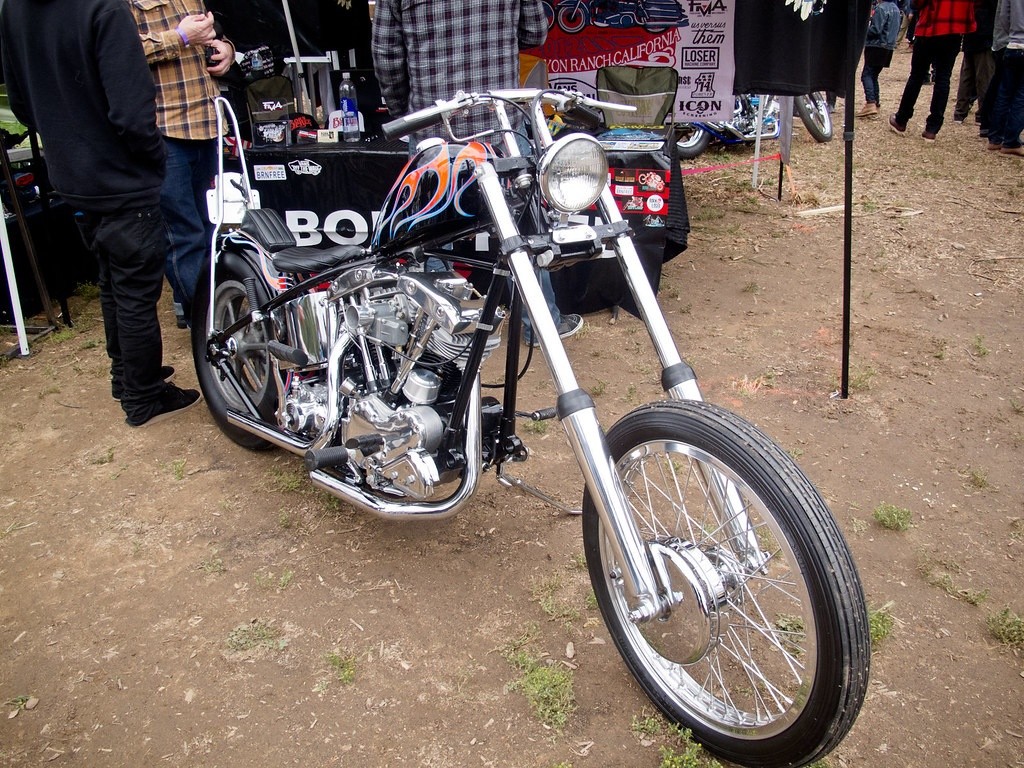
[232,131,691,322]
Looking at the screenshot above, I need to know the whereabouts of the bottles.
[252,50,264,71]
[339,72,361,142]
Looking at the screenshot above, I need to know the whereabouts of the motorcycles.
[189,94,871,768]
[665,90,833,160]
[560,66,690,317]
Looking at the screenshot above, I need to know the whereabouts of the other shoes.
[987,140,1024,156]
[922,76,935,85]
[175,312,190,329]
[953,114,960,120]
[828,104,835,113]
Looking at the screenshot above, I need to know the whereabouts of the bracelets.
[176,27,189,48]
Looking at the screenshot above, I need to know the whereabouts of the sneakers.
[129,382,201,428]
[855,103,878,117]
[111,365,175,402]
[526,314,584,348]
[887,113,906,135]
[920,131,937,142]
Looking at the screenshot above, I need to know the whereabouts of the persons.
[826,0,1024,156]
[0,0,237,429]
[371,0,583,346]
